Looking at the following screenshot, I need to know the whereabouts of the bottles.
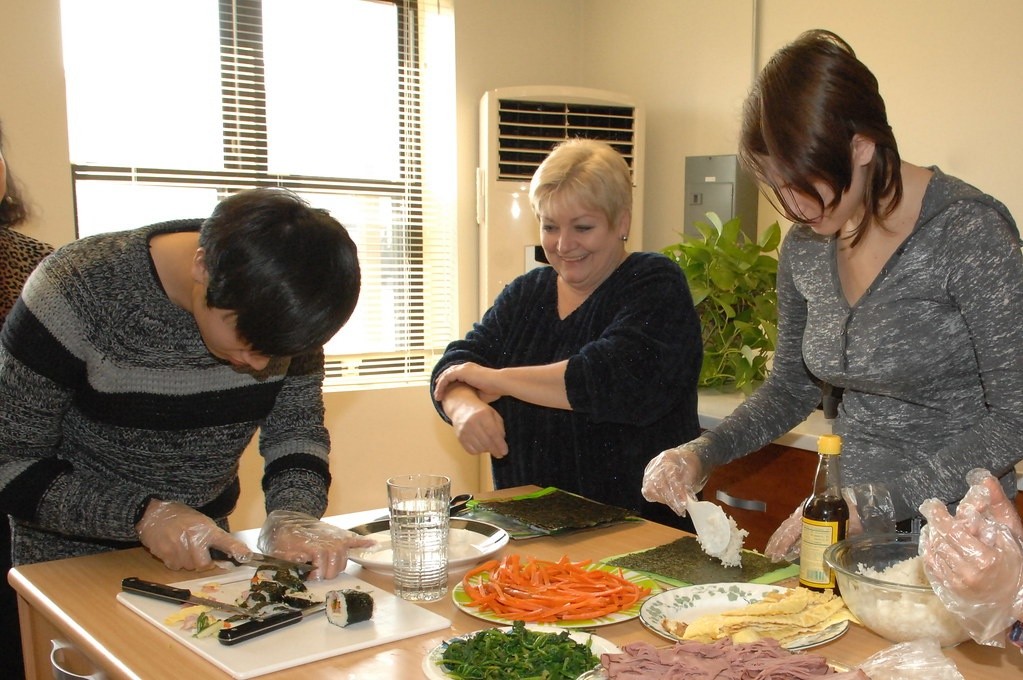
[800,434,849,593]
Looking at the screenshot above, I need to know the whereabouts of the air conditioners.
[473,83,646,492]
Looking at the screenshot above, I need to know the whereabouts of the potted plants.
[660,210,783,397]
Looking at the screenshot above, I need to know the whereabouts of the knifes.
[210,547,318,572]
[122,577,260,619]
[217,590,374,646]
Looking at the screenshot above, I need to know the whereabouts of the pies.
[661,587,862,647]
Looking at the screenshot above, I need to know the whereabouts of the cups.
[386,474,451,602]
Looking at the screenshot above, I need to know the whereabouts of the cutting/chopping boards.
[116,571,452,680]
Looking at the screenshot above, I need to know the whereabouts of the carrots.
[459,554,652,624]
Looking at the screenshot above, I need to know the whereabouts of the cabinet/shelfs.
[8,481,1023,680]
[698,427,827,556]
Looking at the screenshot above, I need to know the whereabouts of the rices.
[855,555,980,652]
[695,506,749,569]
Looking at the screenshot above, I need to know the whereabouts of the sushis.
[224,561,374,629]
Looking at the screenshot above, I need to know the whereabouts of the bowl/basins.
[823,533,971,648]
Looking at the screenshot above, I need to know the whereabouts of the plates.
[452,564,662,628]
[347,518,509,573]
[424,628,621,680]
[640,582,849,652]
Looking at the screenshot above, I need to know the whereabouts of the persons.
[430,139,702,533]
[917,467,1023,656]
[641,30,1023,558]
[1,188,379,680]
[0,144,56,326]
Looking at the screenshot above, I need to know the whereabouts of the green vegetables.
[432,620,601,680]
